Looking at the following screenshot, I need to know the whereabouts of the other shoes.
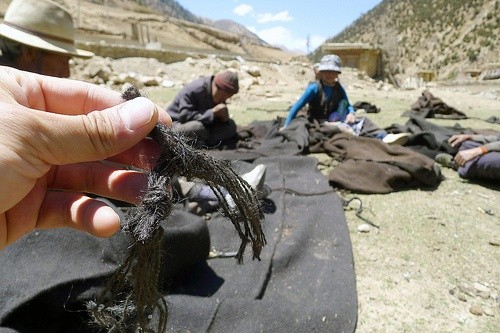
[224,164,273,212]
[382,133,409,146]
[434,153,453,168]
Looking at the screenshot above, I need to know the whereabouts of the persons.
[0,64,173,250]
[164,70,240,150]
[435,130,500,180]
[279,54,410,146]
[0,0,267,214]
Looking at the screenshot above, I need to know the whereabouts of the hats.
[0,0,95,59]
[317,54,343,74]
[214,70,239,95]
